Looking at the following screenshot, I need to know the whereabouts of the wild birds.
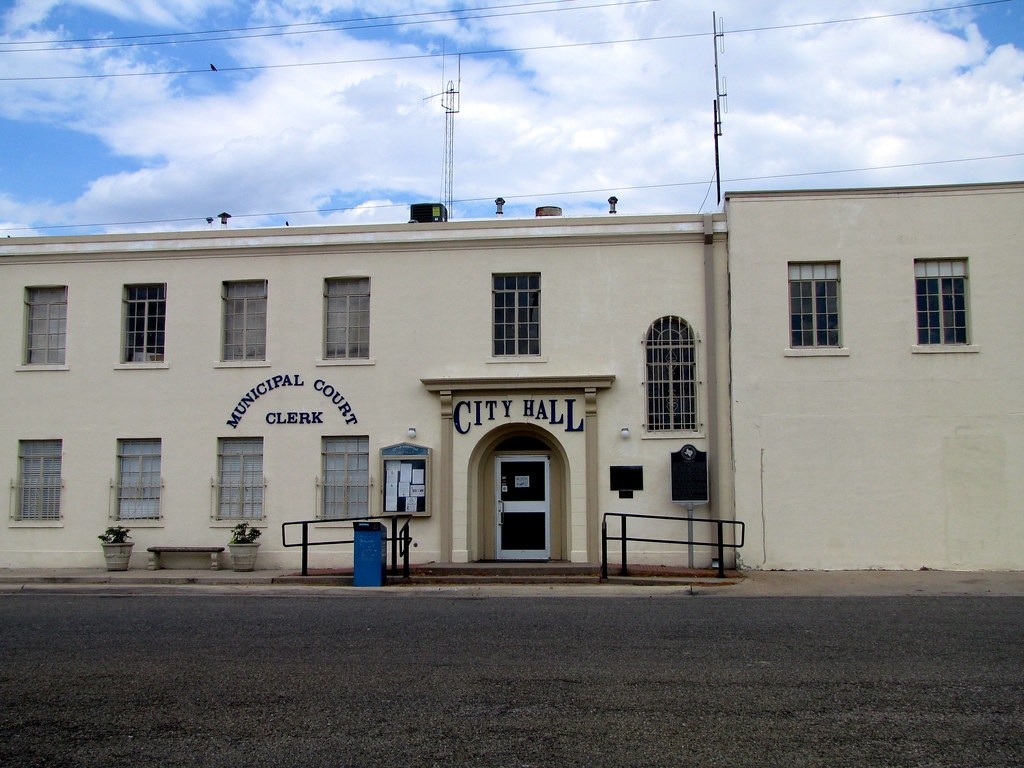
[285,220,290,226]
[208,63,219,71]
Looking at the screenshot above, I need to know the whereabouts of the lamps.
[408,427,416,438]
[620,427,630,438]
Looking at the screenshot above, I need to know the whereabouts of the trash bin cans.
[352,521,388,588]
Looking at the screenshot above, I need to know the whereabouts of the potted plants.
[227,522,262,572]
[97,526,135,572]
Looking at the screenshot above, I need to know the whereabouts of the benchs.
[147,545,225,571]
